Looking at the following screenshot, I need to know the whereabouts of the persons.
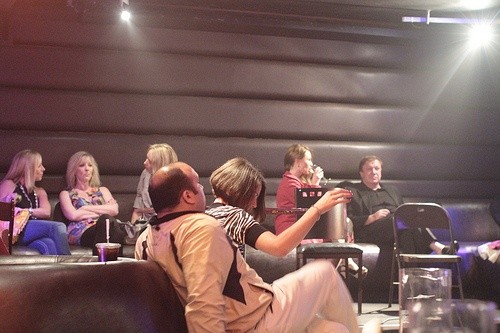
[205,157,353,262]
[345,155,460,296]
[59,151,126,256]
[131,143,180,223]
[274,144,369,279]
[135,162,360,333]
[0,150,71,256]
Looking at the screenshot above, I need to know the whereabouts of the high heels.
[340,265,367,280]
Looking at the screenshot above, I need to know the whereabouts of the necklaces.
[18,183,39,220]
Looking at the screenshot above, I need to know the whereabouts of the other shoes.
[385,289,398,303]
[441,239,459,265]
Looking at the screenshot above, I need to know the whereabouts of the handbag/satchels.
[116,219,137,245]
[12,209,32,245]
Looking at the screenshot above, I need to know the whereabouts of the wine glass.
[309,164,332,186]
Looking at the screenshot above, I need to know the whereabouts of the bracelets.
[313,206,321,221]
[29,208,33,217]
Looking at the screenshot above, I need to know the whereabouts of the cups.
[451,298,496,333]
[398,267,453,333]
[97,243,121,262]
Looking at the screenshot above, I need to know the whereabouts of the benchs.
[12,194,500,303]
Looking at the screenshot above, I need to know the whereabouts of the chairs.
[388,202,465,307]
[294,187,363,314]
